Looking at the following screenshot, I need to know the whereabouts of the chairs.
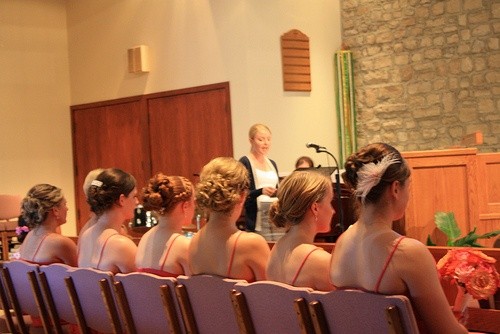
[0,236,500,334]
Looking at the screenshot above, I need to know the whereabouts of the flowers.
[435,249,500,322]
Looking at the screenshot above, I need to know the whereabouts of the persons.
[186,156,275,283]
[239,124,282,235]
[295,157,314,172]
[135,174,194,278]
[17,184,79,334]
[78,167,140,275]
[8,215,38,262]
[264,172,339,293]
[325,142,472,334]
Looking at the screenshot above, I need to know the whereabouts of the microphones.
[306,143,326,149]
